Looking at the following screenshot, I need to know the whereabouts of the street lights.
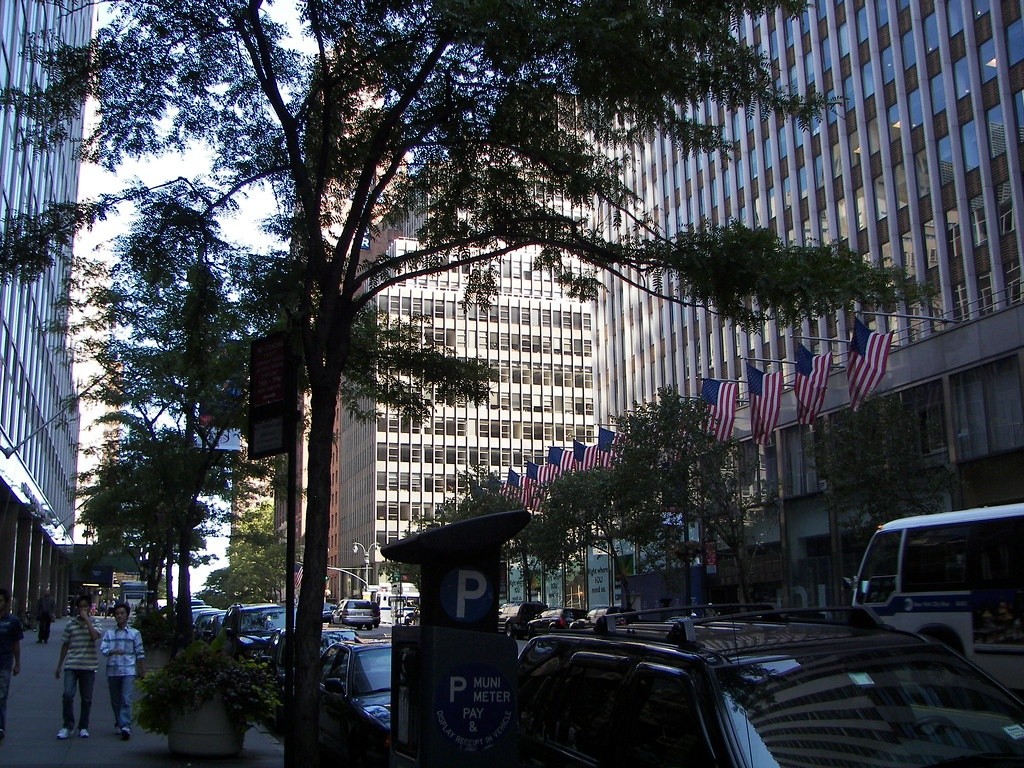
[352,541,381,601]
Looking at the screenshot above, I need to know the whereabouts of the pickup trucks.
[567,607,685,645]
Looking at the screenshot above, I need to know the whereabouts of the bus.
[850,502,1024,698]
[369,590,420,626]
[119,581,148,616]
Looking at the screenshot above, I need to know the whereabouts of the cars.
[524,607,590,640]
[319,639,395,768]
[322,603,337,624]
[157,596,227,649]
[330,598,374,631]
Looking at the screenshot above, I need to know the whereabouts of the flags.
[794,344,833,426]
[573,440,598,472]
[745,363,783,444]
[700,378,738,448]
[547,447,574,480]
[498,461,549,512]
[598,426,624,470]
[847,317,894,412]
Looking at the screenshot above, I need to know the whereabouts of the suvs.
[497,601,550,638]
[218,602,286,665]
[370,601,381,627]
[261,626,365,733]
[519,605,1023,768]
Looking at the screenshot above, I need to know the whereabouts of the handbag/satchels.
[48,601,56,622]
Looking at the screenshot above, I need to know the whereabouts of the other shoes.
[112,726,121,735]
[79,729,89,738]
[36,640,42,643]
[0,727,5,737]
[57,727,74,739]
[122,727,130,739]
[44,640,48,644]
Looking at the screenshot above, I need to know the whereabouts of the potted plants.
[131,604,179,674]
[134,629,283,757]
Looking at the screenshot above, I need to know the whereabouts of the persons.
[100,600,116,616]
[0,588,24,737]
[100,604,146,738]
[55,595,102,739]
[91,601,96,616]
[35,608,56,644]
